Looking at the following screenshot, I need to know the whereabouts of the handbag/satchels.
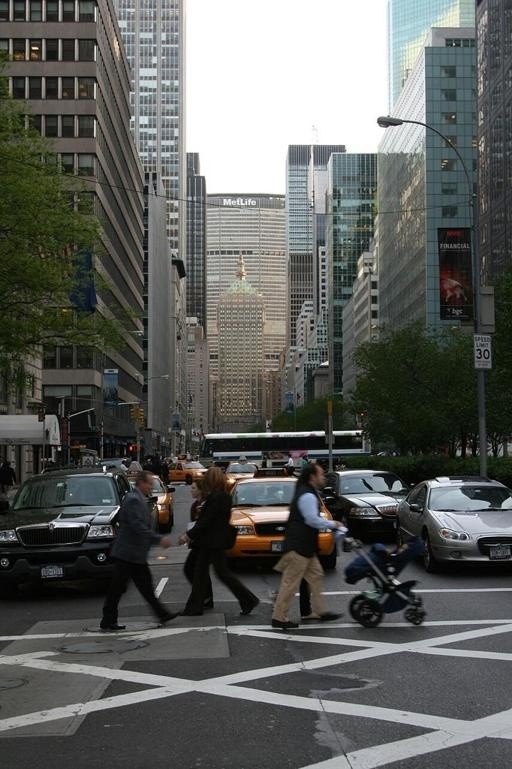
[225,522,240,550]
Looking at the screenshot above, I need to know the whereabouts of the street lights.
[65,407,97,470]
[135,373,169,467]
[266,378,298,431]
[375,114,488,478]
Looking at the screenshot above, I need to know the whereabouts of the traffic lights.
[130,409,135,419]
[129,444,137,458]
[138,419,144,427]
[138,408,143,419]
[360,411,367,427]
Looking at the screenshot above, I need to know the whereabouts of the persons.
[162,459,170,481]
[186,480,214,610]
[269,462,343,630]
[99,471,182,629]
[386,541,411,556]
[1,457,17,486]
[174,465,261,618]
[121,459,128,474]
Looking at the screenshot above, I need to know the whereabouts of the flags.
[436,224,475,321]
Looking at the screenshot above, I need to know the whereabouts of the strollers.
[329,524,426,629]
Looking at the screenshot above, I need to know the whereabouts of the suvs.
[1,464,135,593]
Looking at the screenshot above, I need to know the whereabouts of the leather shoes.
[100,595,338,629]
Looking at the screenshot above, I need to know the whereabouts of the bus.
[197,429,373,473]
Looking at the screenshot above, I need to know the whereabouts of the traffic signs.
[473,336,493,369]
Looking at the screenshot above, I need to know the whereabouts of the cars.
[97,455,211,486]
[124,473,176,533]
[320,469,414,537]
[396,474,511,575]
[219,466,338,572]
[223,454,259,491]
